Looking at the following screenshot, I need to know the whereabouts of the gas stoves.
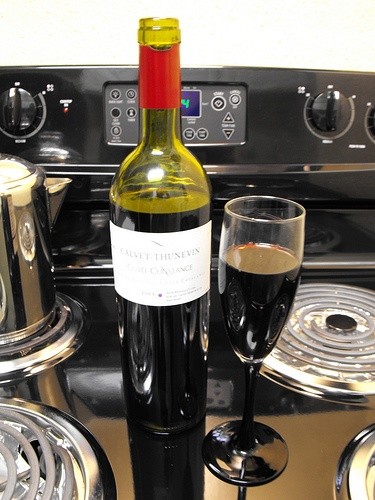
[1,66,375,500]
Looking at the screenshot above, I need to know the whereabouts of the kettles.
[1,152,74,348]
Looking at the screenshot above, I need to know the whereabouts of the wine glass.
[201,195,306,487]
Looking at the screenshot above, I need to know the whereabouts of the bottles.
[105,17,213,439]
[128,420,205,500]
